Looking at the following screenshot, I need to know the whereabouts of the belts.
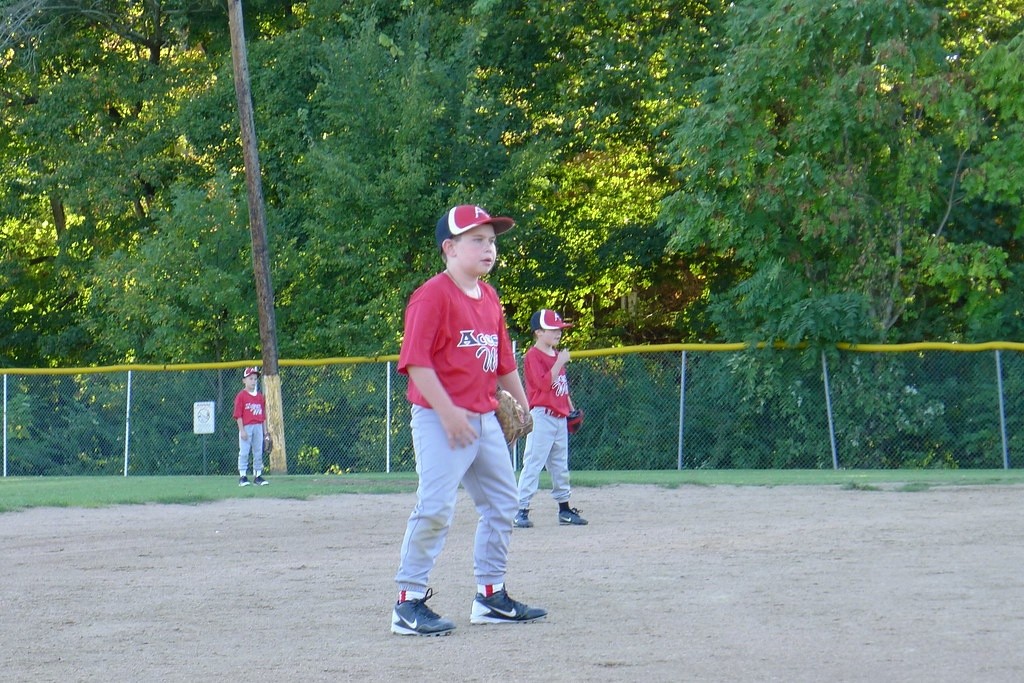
[545,408,566,419]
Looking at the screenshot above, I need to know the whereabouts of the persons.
[233,367,269,486]
[390,205,547,636]
[513,309,588,527]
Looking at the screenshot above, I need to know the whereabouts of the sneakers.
[238,476,251,487]
[471,583,548,624]
[391,587,457,638]
[558,507,589,526]
[512,511,534,527]
[253,476,269,486]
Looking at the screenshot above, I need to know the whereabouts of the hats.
[242,366,261,379]
[530,309,572,333]
[435,204,515,255]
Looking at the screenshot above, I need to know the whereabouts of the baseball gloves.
[263,433,274,454]
[495,385,533,446]
[567,407,583,433]
